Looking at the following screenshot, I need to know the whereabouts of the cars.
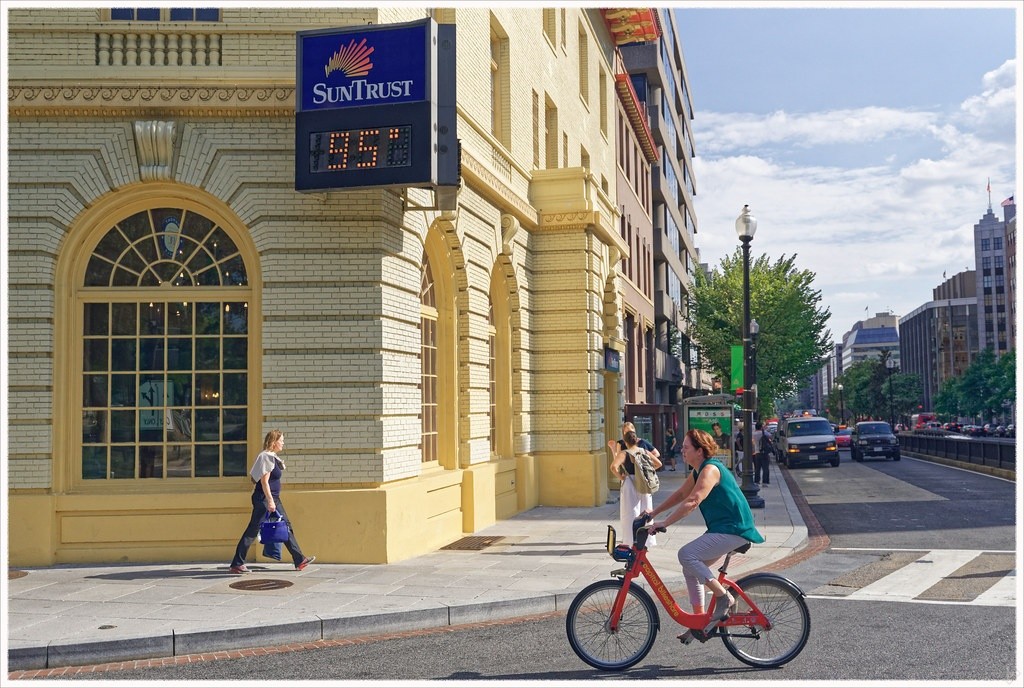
[752,422,778,452]
[927,422,1015,438]
[833,427,853,447]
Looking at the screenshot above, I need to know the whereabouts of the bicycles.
[565,515,812,672]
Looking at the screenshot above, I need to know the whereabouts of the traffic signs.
[140,379,174,432]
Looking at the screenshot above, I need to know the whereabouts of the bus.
[899,413,936,432]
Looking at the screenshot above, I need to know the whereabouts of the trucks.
[793,410,816,418]
[776,416,840,469]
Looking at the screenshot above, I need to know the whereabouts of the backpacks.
[622,448,660,494]
[759,430,776,456]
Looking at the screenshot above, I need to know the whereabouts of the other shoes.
[710,590,736,621]
[676,628,692,639]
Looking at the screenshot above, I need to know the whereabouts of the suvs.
[849,422,901,462]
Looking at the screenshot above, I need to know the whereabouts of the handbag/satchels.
[259,509,289,561]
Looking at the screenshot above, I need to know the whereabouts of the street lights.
[749,318,759,421]
[886,358,894,433]
[736,205,765,508]
[838,383,844,426]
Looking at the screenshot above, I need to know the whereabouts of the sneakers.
[297,556,316,572]
[230,565,252,573]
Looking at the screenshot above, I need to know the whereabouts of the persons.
[736,422,771,487]
[607,421,663,548]
[641,428,767,641]
[230,428,317,574]
[666,428,676,471]
[711,422,730,449]
